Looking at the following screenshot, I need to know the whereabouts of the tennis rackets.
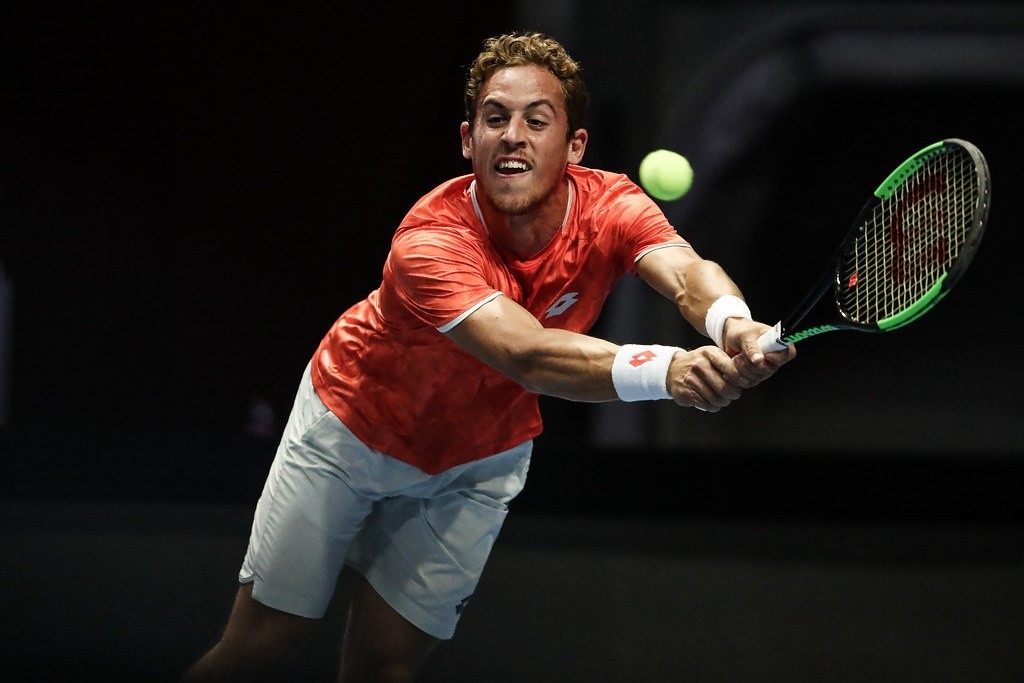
[697,137,991,411]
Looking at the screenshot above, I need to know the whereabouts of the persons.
[181,31,798,683]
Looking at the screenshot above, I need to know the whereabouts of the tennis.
[640,149,693,204]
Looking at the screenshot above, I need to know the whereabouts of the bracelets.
[705,296,752,351]
[612,345,687,401]
